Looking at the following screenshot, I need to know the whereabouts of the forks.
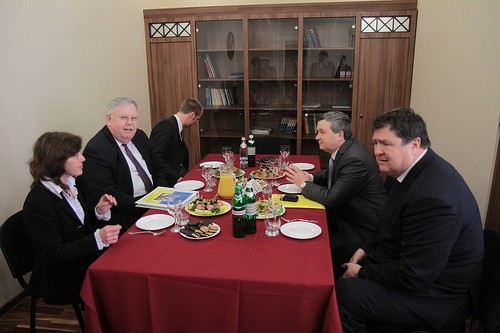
[281,217,318,223]
[128,229,166,236]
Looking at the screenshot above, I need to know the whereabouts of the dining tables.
[82,153,341,333]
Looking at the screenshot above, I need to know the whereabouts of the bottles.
[243,183,257,234]
[231,185,247,238]
[239,137,249,169]
[246,134,256,167]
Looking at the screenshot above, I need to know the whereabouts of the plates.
[199,160,225,168]
[250,170,285,180]
[135,213,175,231]
[277,183,302,193]
[255,200,286,220]
[289,162,315,170]
[280,221,322,239]
[173,179,204,191]
[179,222,221,240]
[184,200,231,217]
[212,168,245,178]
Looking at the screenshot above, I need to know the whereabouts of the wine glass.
[201,164,213,193]
[280,145,290,170]
[167,196,182,233]
[267,194,283,220]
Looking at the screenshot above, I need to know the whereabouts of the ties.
[328,157,334,189]
[122,144,153,193]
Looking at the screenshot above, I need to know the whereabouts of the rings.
[114,236,115,240]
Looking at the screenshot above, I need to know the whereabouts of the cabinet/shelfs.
[142,0,419,177]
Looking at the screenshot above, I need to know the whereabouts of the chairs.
[463,228,500,333]
[0,210,86,333]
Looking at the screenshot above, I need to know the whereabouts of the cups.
[217,164,236,199]
[221,146,235,165]
[263,211,281,237]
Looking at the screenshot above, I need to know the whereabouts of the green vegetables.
[251,170,270,177]
[257,205,265,213]
[189,196,221,214]
[235,169,242,175]
[256,156,277,164]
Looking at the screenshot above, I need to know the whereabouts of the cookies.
[180,220,218,238]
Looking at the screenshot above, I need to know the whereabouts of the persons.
[285,111,387,280]
[335,107,484,333]
[149,98,203,188]
[22,132,122,302]
[75,96,158,238]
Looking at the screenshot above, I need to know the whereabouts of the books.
[204,53,220,78]
[303,113,324,134]
[279,117,297,135]
[252,127,272,135]
[304,25,320,48]
[227,73,244,79]
[206,87,238,106]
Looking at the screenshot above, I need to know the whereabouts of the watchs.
[300,181,310,190]
